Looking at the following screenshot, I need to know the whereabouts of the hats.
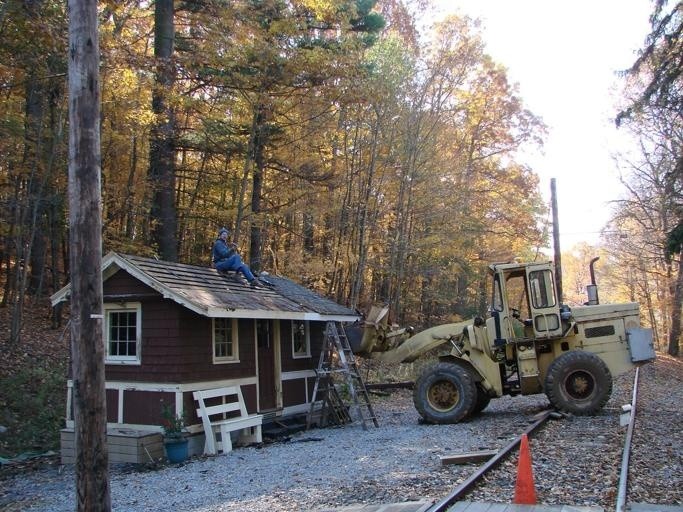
[220,229,228,235]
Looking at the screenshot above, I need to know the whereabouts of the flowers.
[156,398,191,438]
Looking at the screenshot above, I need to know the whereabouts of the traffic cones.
[510,433,539,506]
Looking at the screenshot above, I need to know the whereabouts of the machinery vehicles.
[341,254,656,424]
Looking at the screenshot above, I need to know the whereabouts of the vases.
[163,433,189,464]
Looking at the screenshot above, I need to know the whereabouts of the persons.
[212,228,265,287]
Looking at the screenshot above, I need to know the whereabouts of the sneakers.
[251,281,264,288]
[234,274,248,284]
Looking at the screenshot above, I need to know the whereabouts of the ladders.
[306,320,379,431]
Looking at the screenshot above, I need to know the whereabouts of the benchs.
[192,385,263,456]
